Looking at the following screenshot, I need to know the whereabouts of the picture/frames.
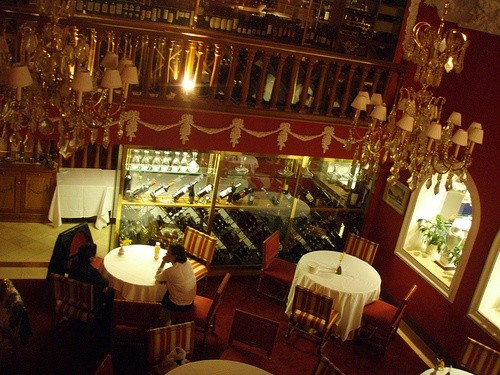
[382,178,411,216]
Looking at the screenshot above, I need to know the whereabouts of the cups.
[308,262,320,273]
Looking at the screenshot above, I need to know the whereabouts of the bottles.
[347,182,362,209]
[277,160,292,178]
[263,211,359,263]
[314,186,335,206]
[305,20,335,49]
[327,164,359,183]
[261,187,281,206]
[120,207,165,245]
[154,242,160,260]
[150,182,174,201]
[300,185,317,206]
[218,183,243,199]
[236,156,248,175]
[301,162,314,179]
[266,12,303,47]
[282,188,294,203]
[228,187,255,202]
[164,206,280,266]
[75,0,194,25]
[197,184,214,199]
[173,181,198,201]
[208,8,267,43]
[124,172,155,201]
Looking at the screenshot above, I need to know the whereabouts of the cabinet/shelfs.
[119,143,376,269]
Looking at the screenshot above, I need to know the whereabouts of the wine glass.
[130,150,199,173]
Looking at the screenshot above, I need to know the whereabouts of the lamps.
[0,0,141,168]
[341,0,483,195]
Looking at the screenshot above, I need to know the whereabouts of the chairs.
[0,225,500,375]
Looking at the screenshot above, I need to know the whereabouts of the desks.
[47,167,122,230]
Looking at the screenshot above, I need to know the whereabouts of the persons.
[71,244,115,326]
[155,245,196,311]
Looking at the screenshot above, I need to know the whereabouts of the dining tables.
[241,191,311,218]
[285,249,382,342]
[419,367,474,375]
[164,359,274,375]
[102,244,174,304]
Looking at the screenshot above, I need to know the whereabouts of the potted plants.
[417,214,450,255]
